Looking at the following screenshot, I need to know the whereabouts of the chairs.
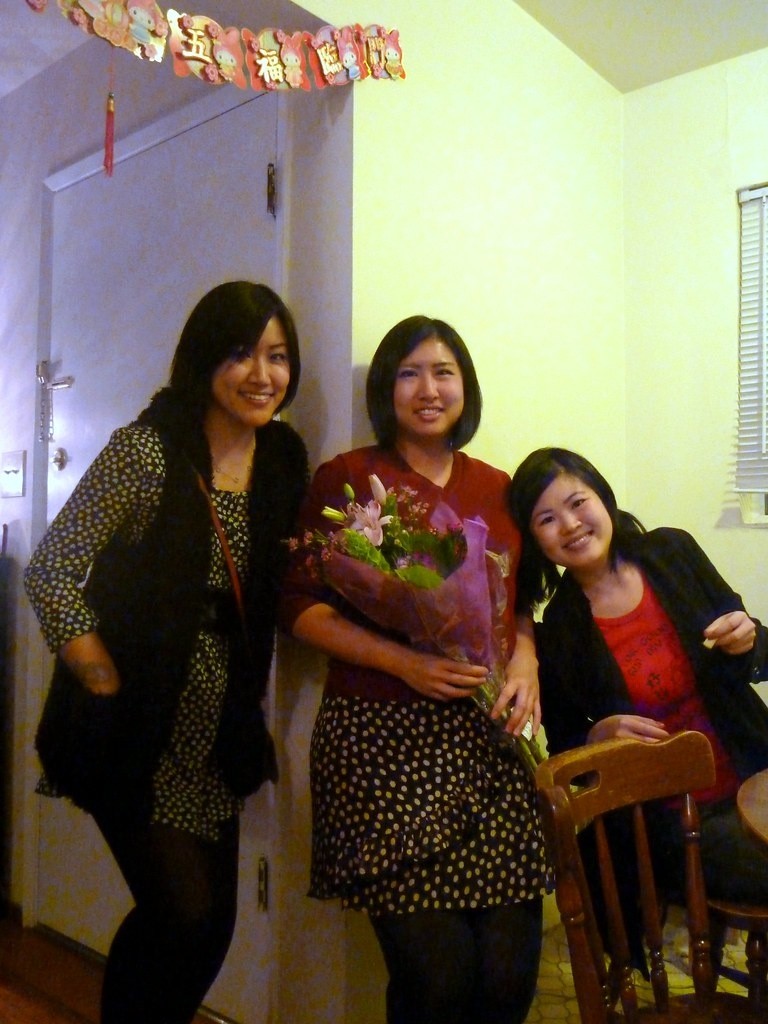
[528,733,768,1024]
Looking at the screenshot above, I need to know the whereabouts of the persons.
[510,448,768,981]
[269,317,557,1023]
[21,277,311,1023]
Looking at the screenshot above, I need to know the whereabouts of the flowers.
[277,472,584,838]
